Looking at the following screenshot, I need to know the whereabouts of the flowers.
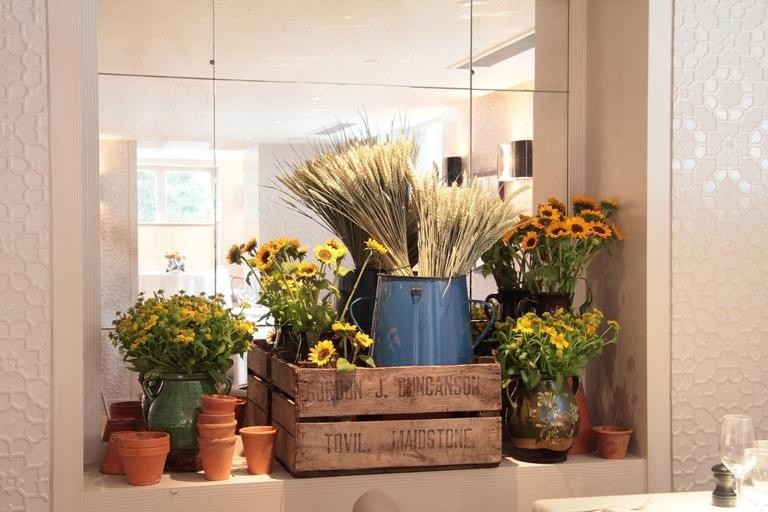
[108,290,258,389]
[225,229,390,371]
[476,192,626,388]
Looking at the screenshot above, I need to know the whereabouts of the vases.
[100,372,277,487]
[349,268,498,367]
[505,293,637,462]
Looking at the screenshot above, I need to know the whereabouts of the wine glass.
[720,413,768,509]
